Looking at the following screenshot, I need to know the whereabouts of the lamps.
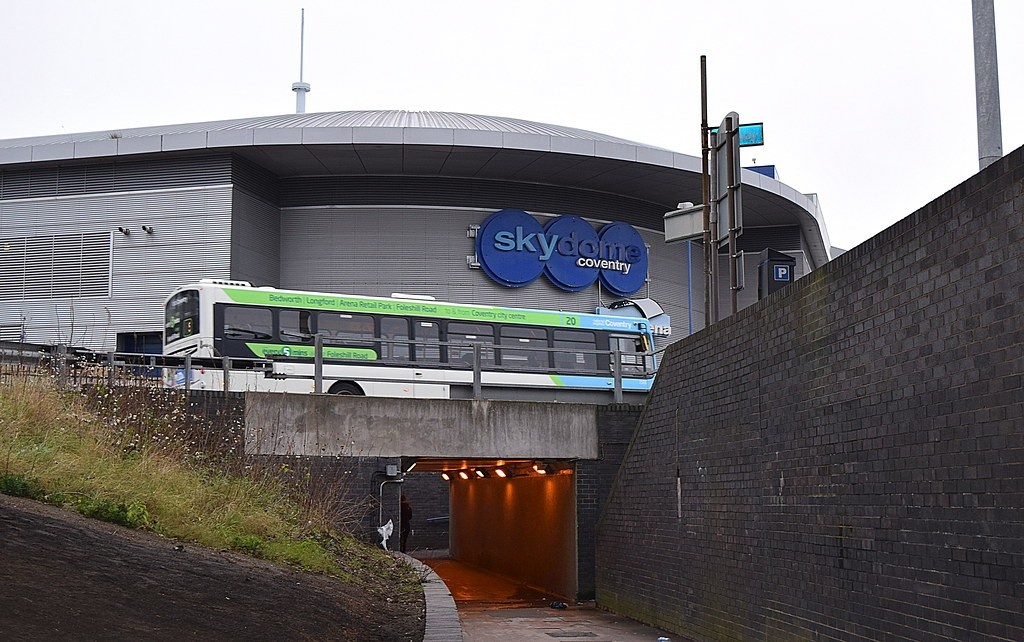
[475,468,491,479]
[494,467,511,477]
[532,464,553,475]
[440,471,454,482]
[458,471,472,480]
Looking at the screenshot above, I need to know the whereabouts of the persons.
[400,495,412,553]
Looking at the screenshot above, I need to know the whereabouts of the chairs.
[462,353,474,364]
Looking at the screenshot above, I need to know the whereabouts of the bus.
[161,277,659,400]
